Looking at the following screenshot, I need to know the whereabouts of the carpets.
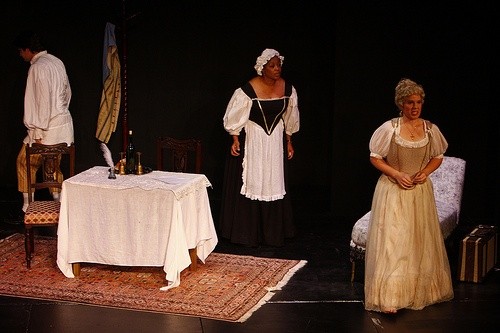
[0,233,308,323]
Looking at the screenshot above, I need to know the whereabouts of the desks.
[56,166,218,291]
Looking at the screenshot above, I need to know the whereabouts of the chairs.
[24,142,75,269]
[141,136,201,175]
[350,157,467,282]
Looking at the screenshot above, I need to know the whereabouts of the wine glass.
[136,152,143,174]
[118,152,125,174]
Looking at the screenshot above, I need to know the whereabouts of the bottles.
[126,130,135,174]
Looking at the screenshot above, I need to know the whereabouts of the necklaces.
[401,121,421,141]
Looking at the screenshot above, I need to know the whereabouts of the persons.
[15,36,75,218]
[369,80,444,315]
[222,47,300,246]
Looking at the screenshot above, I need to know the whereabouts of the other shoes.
[4,209,33,225]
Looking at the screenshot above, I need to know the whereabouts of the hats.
[254,46,285,76]
